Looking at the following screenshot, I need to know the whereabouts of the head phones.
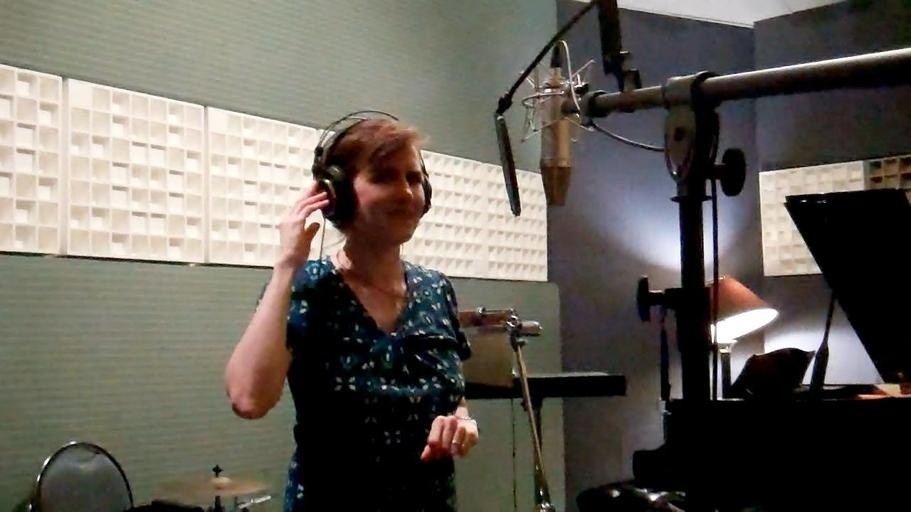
[312,110,431,221]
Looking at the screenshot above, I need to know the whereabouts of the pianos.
[632,188,911,510]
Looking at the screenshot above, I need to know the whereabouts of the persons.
[224,110,479,512]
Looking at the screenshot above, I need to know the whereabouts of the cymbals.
[157,477,269,496]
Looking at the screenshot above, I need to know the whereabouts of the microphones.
[541,44,573,205]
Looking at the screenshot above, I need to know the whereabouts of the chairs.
[24,441,203,512]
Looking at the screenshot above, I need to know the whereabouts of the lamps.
[636,272,781,346]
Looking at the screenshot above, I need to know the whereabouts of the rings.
[451,440,461,446]
